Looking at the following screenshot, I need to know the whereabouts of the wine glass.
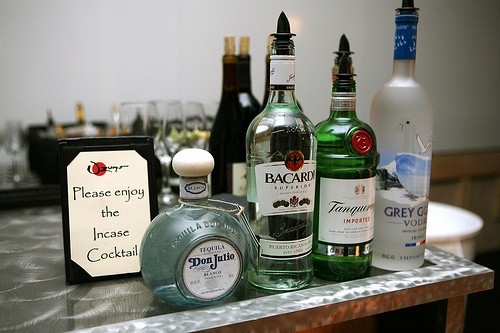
[114,99,218,205]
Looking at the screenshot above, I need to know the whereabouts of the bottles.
[370,0,433,272]
[245,11,318,292]
[262,33,305,116]
[314,33,380,283]
[209,35,262,197]
[138,149,252,312]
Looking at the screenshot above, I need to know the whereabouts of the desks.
[0,203,494,333]
